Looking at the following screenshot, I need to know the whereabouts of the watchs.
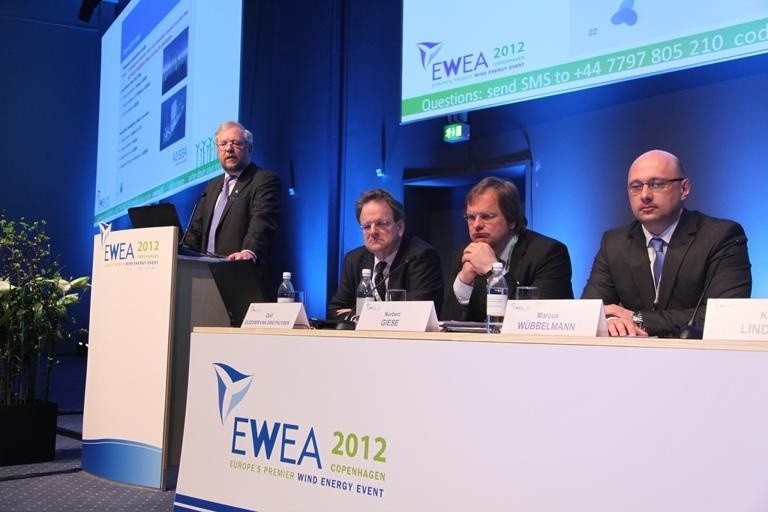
[632,310,643,329]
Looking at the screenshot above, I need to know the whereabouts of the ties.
[206,175,236,257]
[375,260,387,301]
[649,236,665,290]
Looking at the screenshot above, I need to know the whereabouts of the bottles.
[486,262,508,334]
[355,269,375,331]
[277,272,295,303]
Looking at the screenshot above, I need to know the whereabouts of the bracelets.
[482,267,494,277]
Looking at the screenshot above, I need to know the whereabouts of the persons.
[184,121,287,303]
[326,188,443,324]
[581,149,752,339]
[450,176,575,323]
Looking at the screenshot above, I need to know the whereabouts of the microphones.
[178,192,208,248]
[335,253,417,330]
[672,236,749,339]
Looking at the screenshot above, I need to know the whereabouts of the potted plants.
[0,208,92,468]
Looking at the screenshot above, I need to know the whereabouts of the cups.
[385,289,406,301]
[516,286,540,299]
[289,291,305,308]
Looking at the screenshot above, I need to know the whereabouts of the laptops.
[129,204,209,256]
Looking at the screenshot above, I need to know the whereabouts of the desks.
[172,327,768,512]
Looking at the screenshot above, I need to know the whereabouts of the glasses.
[216,141,246,149]
[463,212,497,224]
[627,178,685,196]
[359,220,389,232]
